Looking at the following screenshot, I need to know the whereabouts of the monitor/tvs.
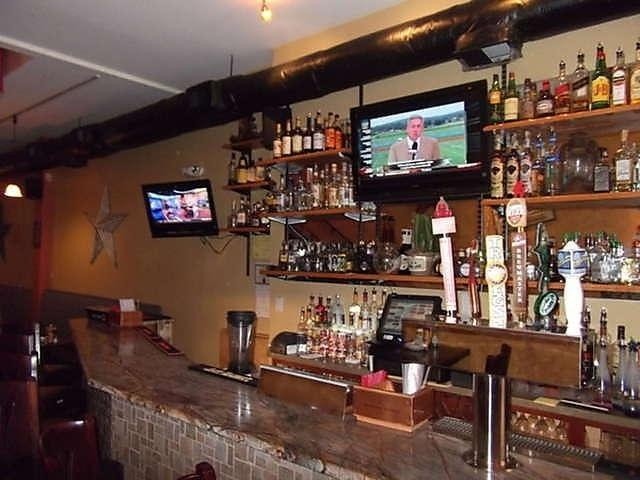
[141,179,219,238]
[378,293,442,343]
[350,78,490,205]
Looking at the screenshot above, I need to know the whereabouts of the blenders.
[225,310,255,375]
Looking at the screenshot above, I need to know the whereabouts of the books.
[388,158,436,172]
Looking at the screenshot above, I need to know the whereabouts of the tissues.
[112,297,144,329]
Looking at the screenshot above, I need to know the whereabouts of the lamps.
[0,75,101,198]
[454,14,523,70]
[259,0,272,23]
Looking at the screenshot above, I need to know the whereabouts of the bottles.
[226,196,270,228]
[229,152,268,183]
[484,40,640,127]
[583,309,640,401]
[295,287,385,365]
[489,122,640,194]
[271,111,344,158]
[536,230,639,283]
[283,162,359,207]
[278,215,400,275]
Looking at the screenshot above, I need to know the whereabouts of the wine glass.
[509,408,571,445]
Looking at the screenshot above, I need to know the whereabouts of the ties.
[412,142,417,160]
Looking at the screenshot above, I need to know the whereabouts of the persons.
[158,194,211,221]
[386,113,442,163]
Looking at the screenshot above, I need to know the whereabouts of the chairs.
[1,321,217,480]
